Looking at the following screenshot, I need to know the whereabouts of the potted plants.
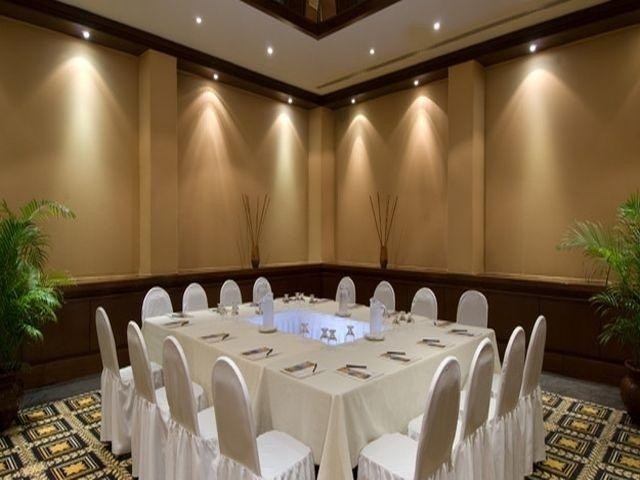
[556,187,640,428]
[0,196,80,435]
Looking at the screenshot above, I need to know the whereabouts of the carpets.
[0,388,640,480]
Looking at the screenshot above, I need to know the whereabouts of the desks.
[138,291,502,480]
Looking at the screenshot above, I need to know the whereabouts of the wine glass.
[344,325,355,342]
[328,329,337,344]
[283,292,315,304]
[298,322,312,338]
[320,328,330,343]
[217,301,239,318]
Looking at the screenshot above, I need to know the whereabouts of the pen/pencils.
[452,329,467,332]
[266,348,273,355]
[423,339,440,342]
[222,333,229,340]
[181,320,188,326]
[312,363,318,372]
[386,351,406,355]
[345,364,367,369]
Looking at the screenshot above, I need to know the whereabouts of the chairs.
[454,289,491,331]
[127,321,207,480]
[252,279,274,304]
[408,337,497,480]
[158,334,218,480]
[141,285,174,319]
[334,277,358,305]
[210,355,317,479]
[373,281,396,313]
[492,315,550,478]
[180,281,210,313]
[219,278,244,308]
[454,325,525,479]
[96,306,162,460]
[409,287,439,318]
[353,355,463,479]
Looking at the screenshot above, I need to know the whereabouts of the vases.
[380,246,388,269]
[251,247,260,273]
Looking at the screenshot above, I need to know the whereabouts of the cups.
[337,285,352,315]
[369,298,388,337]
[262,293,274,329]
[392,310,414,326]
